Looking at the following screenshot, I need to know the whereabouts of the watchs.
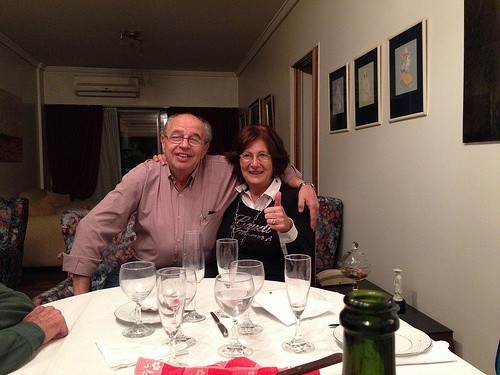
[299,181,316,189]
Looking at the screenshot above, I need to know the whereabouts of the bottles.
[339,288,400,375]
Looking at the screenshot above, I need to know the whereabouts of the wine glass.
[215,239,239,318]
[213,272,255,359]
[229,259,265,336]
[281,254,316,354]
[158,269,190,368]
[339,248,373,291]
[118,261,155,339]
[182,231,205,323]
[156,267,197,350]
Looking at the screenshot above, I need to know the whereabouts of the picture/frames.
[239,112,247,130]
[263,93,274,127]
[353,45,381,129]
[389,18,427,122]
[249,97,261,125]
[327,61,349,134]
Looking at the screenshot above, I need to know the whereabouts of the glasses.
[238,151,273,162]
[161,132,206,147]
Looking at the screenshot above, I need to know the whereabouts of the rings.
[272,219,275,225]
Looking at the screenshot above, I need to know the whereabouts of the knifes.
[210,311,228,338]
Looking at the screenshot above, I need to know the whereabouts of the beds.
[20,189,99,267]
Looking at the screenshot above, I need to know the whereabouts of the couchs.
[32,198,342,307]
[0,197,28,282]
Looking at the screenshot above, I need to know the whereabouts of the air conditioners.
[72,76,141,98]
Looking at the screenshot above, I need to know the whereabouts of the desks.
[5,278,485,375]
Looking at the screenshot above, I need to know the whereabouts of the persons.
[57,113,319,296]
[0,282,68,375]
[152,123,315,287]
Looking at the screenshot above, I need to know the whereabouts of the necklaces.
[230,195,270,252]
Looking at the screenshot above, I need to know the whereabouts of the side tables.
[323,270,453,349]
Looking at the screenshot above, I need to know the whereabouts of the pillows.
[17,189,72,214]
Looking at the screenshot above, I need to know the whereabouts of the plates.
[333,324,433,357]
[113,297,161,324]
[252,287,325,312]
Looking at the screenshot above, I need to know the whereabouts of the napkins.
[394,341,458,368]
[94,340,190,372]
